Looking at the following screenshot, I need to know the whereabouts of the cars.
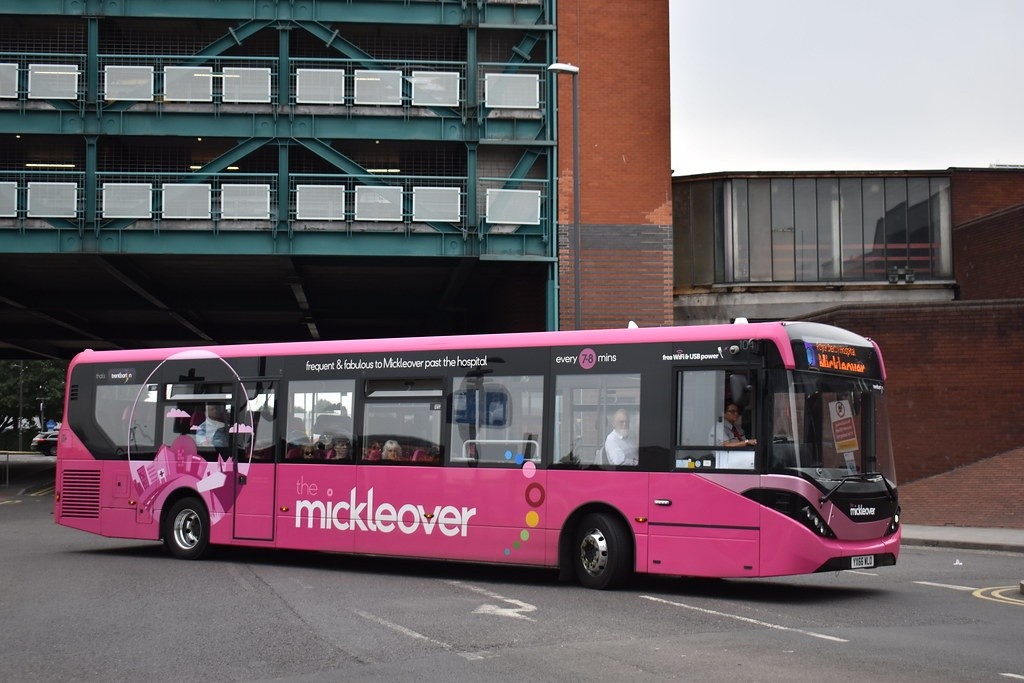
[30,431,59,456]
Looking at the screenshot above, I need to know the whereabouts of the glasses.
[304,451,314,456]
[726,410,739,414]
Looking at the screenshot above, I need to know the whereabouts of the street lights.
[35,397,50,432]
[547,60,581,457]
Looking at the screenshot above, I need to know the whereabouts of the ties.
[732,424,744,441]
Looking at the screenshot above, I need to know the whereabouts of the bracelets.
[744,439,749,445]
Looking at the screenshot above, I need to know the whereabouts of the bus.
[54,318,902,593]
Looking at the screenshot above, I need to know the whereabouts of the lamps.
[889,274,899,282]
[905,274,916,282]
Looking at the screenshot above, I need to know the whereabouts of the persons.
[708,400,757,447]
[195,403,229,447]
[381,439,402,461]
[46,418,62,432]
[300,434,351,460]
[604,408,638,466]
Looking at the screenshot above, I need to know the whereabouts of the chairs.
[594,448,608,465]
[120,403,435,462]
